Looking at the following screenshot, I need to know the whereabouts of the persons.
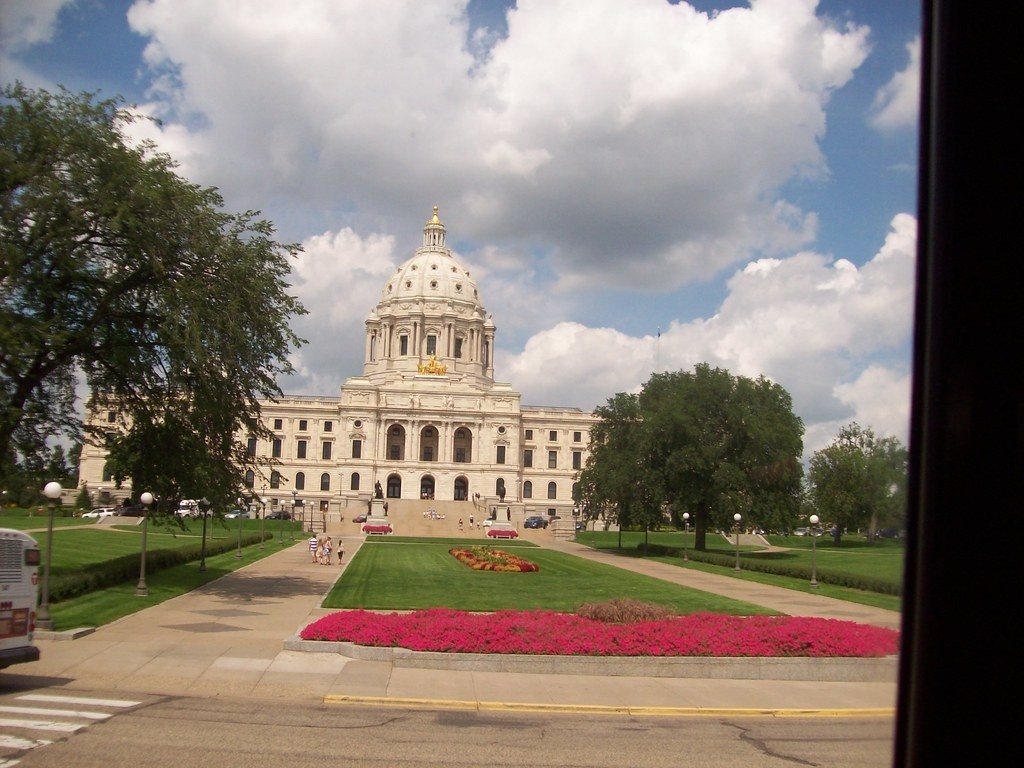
[475,493,480,500]
[375,480,382,498]
[507,507,510,521]
[469,513,474,529]
[492,507,496,520]
[500,483,506,499]
[336,540,345,564]
[457,518,463,532]
[383,501,389,516]
[476,520,480,529]
[309,535,333,565]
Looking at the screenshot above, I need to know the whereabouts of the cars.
[266,510,291,520]
[810,527,825,537]
[575,520,587,531]
[830,528,842,537]
[352,514,368,523]
[793,528,807,537]
[225,509,250,520]
[876,527,899,538]
[82,506,118,519]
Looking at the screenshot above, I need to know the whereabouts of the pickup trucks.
[523,515,548,529]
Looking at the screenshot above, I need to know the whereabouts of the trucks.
[0,527,42,669]
[177,500,215,519]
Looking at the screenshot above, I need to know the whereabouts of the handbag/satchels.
[327,547,332,552]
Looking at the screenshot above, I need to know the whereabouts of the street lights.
[259,498,267,549]
[98,486,103,508]
[261,485,268,497]
[234,498,245,561]
[277,500,286,545]
[302,498,306,535]
[309,501,314,532]
[292,487,298,502]
[35,481,64,629]
[734,513,742,571]
[288,499,296,541]
[135,492,154,595]
[810,513,820,589]
[196,496,211,574]
[682,513,689,563]
[515,477,521,503]
[339,470,344,496]
